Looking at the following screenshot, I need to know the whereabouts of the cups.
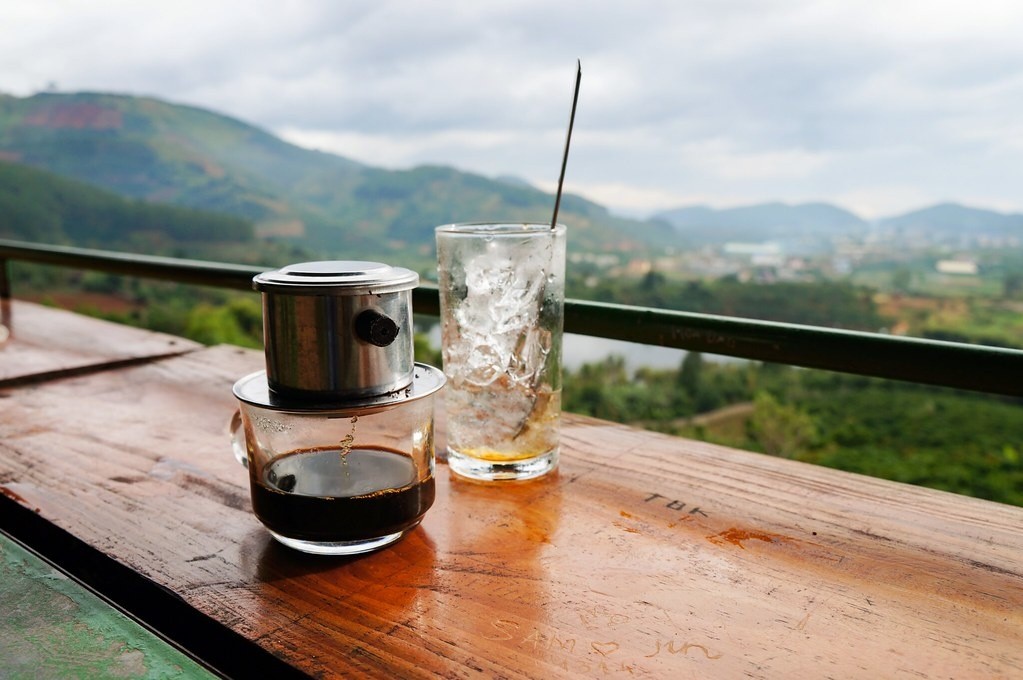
[435,225,565,484]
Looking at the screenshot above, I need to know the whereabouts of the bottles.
[227,361,445,558]
